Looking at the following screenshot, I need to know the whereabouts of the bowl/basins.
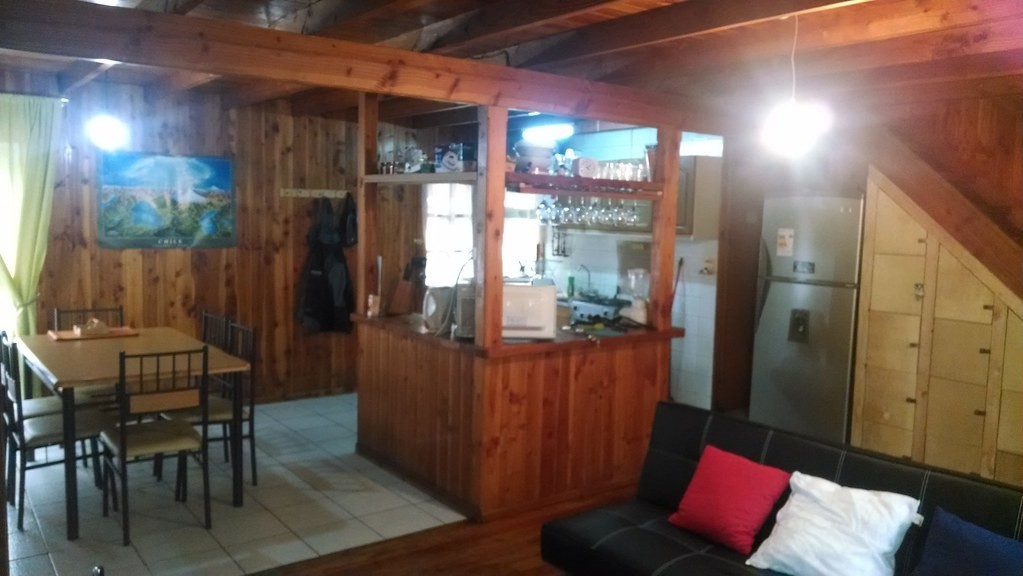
[515,144,554,158]
[516,156,553,172]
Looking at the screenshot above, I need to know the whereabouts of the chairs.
[1,330,93,502]
[173,308,232,465]
[160,318,259,503]
[97,345,211,545]
[1,338,118,533]
[51,306,124,466]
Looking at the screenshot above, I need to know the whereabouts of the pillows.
[912,505,1023,576]
[744,469,922,576]
[668,446,792,554]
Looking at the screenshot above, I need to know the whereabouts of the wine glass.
[537,192,640,225]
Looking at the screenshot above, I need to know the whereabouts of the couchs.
[540,400,1023,576]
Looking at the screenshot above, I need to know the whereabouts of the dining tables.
[15,325,252,539]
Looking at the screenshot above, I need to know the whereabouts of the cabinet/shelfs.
[850,177,1022,489]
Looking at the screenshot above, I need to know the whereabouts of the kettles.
[422,286,454,334]
